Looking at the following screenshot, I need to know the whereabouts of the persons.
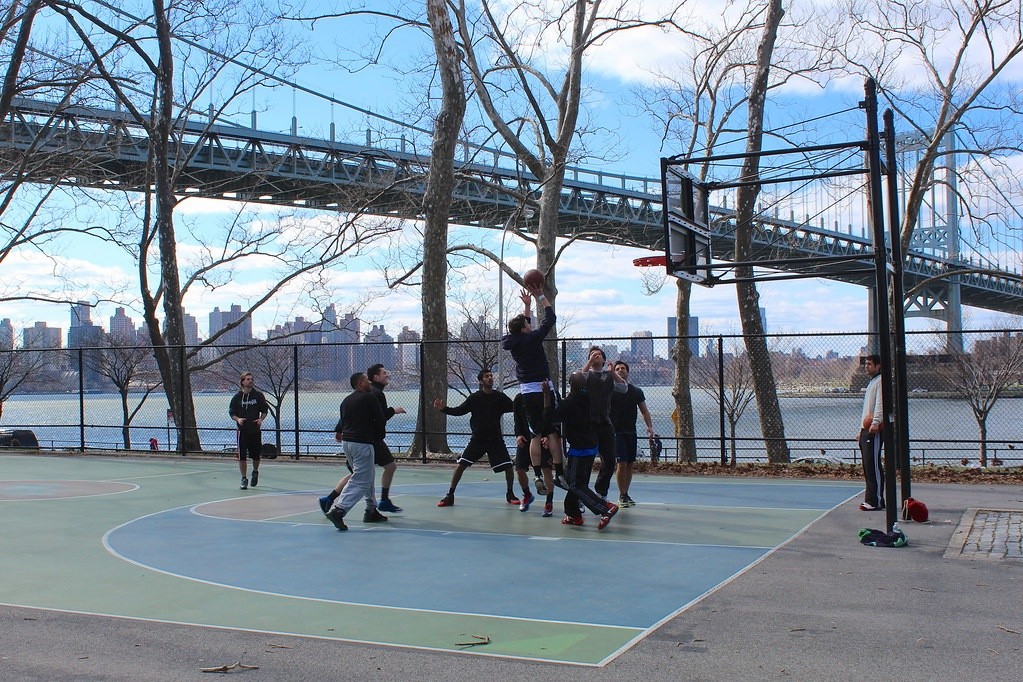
[320,364,406,512]
[433,370,520,506]
[325,372,388,530]
[609,361,654,507]
[502,284,627,531]
[855,355,884,510]
[229,373,268,490]
[649,434,662,461]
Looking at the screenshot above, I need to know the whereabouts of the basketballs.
[523,268,545,289]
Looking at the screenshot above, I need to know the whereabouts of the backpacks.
[901,498,928,523]
[261,443,277,460]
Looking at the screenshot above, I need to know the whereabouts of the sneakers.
[319,497,331,513]
[326,510,348,531]
[561,515,583,526]
[534,477,547,495]
[378,498,402,512]
[543,502,553,517]
[506,493,520,505]
[363,509,387,522]
[519,494,534,511]
[553,475,570,491]
[598,503,619,530]
[438,493,454,506]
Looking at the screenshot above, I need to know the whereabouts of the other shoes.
[250,470,259,487]
[628,495,636,506]
[859,502,874,512]
[600,495,611,502]
[240,477,249,489]
[618,494,629,508]
[578,499,586,512]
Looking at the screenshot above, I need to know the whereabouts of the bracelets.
[539,295,544,300]
